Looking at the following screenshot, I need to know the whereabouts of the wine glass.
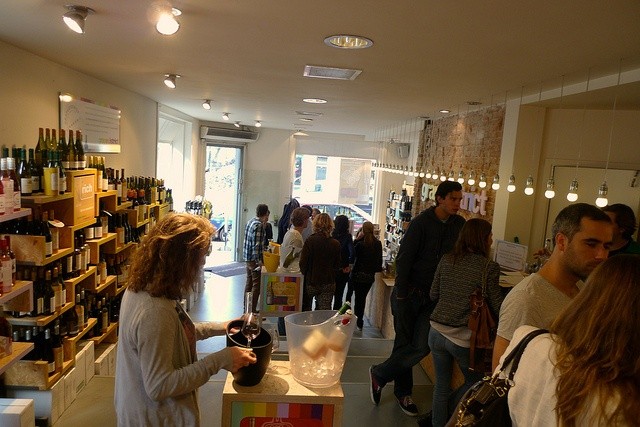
[240,312,263,356]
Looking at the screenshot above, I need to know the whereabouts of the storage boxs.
[76,340,95,385]
[62,365,76,409]
[74,348,86,395]
[6,373,65,426]
[97,342,116,375]
[0,397,35,426]
[94,342,108,375]
[180,270,204,313]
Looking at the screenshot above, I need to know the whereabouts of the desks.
[222,359,344,426]
[259,265,304,354]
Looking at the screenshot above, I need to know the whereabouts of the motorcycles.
[208,213,234,242]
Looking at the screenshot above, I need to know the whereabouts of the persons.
[532,203,640,282]
[290,205,313,243]
[311,208,321,220]
[351,221,382,328]
[263,210,274,245]
[113,211,258,427]
[491,203,613,375]
[333,215,354,309]
[277,199,300,244]
[369,180,467,417]
[416,218,504,427]
[242,204,268,314]
[346,228,364,304]
[493,244,640,427]
[279,206,311,335]
[300,212,340,310]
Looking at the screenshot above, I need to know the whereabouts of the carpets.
[203,260,247,277]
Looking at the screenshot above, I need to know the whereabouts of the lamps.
[369,113,431,178]
[595,57,623,206]
[479,94,493,187]
[491,91,507,189]
[448,104,460,181]
[566,68,591,202]
[222,112,231,120]
[524,81,542,195]
[157,8,183,34]
[61,4,96,33]
[507,85,524,191]
[163,73,182,88]
[234,121,241,127]
[432,115,444,179]
[202,99,213,109]
[468,97,482,185]
[457,102,470,185]
[254,120,262,127]
[440,110,452,181]
[542,80,564,199]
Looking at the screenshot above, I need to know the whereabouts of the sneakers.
[398,394,419,416]
[369,365,382,405]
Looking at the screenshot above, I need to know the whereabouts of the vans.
[300,201,375,236]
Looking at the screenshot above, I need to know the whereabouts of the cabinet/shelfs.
[0,166,171,390]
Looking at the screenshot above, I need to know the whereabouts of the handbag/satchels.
[443,376,514,427]
[469,288,496,373]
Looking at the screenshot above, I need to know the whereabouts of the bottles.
[97,267,101,287]
[0,217,22,234]
[72,237,82,278]
[57,263,67,308]
[13,325,45,360]
[3,235,17,286]
[127,175,167,209]
[166,188,173,212]
[45,128,53,151]
[107,250,131,288]
[240,292,261,324]
[94,216,103,239]
[101,156,109,192]
[49,267,63,313]
[84,154,100,168]
[112,169,123,206]
[92,301,103,336]
[293,249,304,260]
[0,304,13,360]
[23,211,34,235]
[41,269,56,316]
[67,307,79,337]
[38,149,48,193]
[80,235,88,274]
[115,215,125,248]
[26,271,42,317]
[51,325,64,373]
[51,128,58,151]
[266,281,275,306]
[74,130,86,170]
[41,329,56,378]
[35,127,48,174]
[1,157,15,214]
[48,208,60,254]
[39,210,53,258]
[6,157,22,212]
[16,147,33,196]
[43,151,60,196]
[61,254,73,280]
[57,150,69,195]
[74,294,84,332]
[539,238,553,271]
[323,309,353,362]
[27,148,40,193]
[0,238,14,293]
[9,268,29,319]
[99,202,109,238]
[0,180,6,215]
[101,298,109,333]
[108,168,115,189]
[92,297,97,312]
[57,128,70,170]
[120,168,128,203]
[80,291,89,329]
[120,213,129,244]
[282,247,296,268]
[3,147,10,157]
[83,241,91,271]
[84,224,94,240]
[0,258,4,297]
[264,329,281,372]
[185,199,204,216]
[301,301,353,362]
[12,147,20,173]
[67,129,79,170]
[99,258,108,284]
[84,304,94,339]
[58,315,69,337]
[124,213,133,241]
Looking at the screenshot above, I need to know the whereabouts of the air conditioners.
[200,125,259,142]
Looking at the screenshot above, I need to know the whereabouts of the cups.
[520,262,535,278]
